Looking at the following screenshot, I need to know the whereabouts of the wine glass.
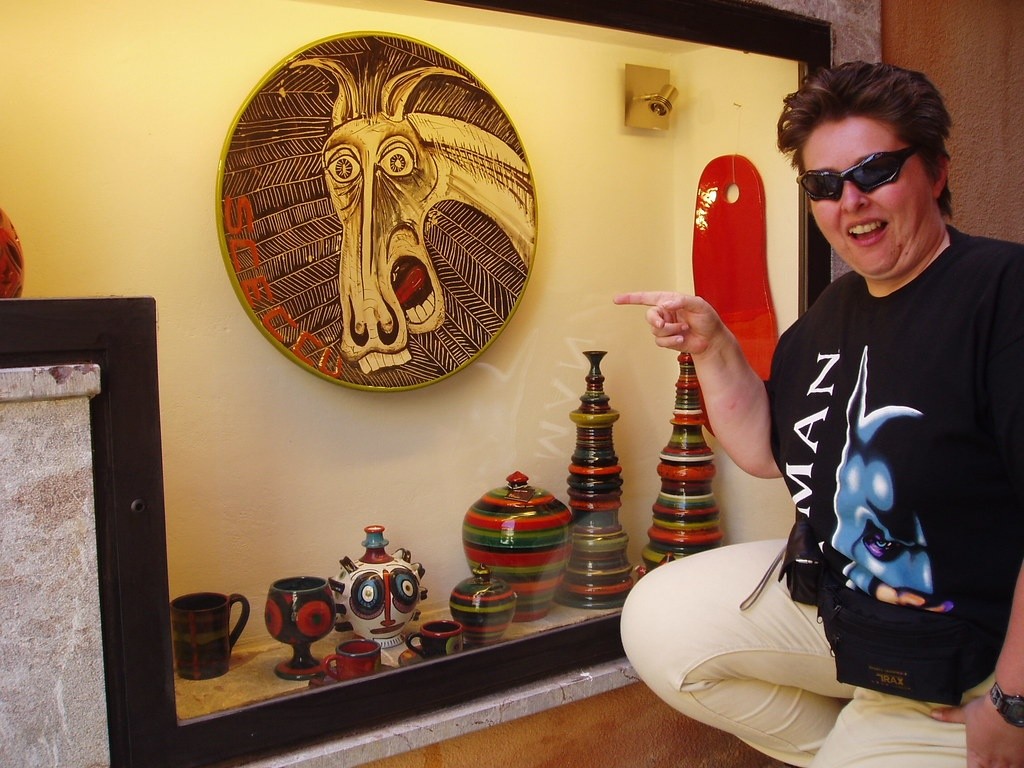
[265,575,336,679]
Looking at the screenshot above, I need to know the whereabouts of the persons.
[612,61,1024,768]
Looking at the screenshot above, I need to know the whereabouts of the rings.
[1012,761,1024,768]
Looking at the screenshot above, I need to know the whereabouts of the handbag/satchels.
[812,565,1014,707]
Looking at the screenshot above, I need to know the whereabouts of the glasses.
[796,146,919,202]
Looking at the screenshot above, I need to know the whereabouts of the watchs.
[989,682,1024,727]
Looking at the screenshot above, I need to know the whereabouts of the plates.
[308,664,395,688]
[398,644,472,667]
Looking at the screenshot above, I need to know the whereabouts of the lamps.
[624,62,679,131]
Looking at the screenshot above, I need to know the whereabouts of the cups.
[169,593,250,679]
[322,639,381,682]
[405,620,464,659]
